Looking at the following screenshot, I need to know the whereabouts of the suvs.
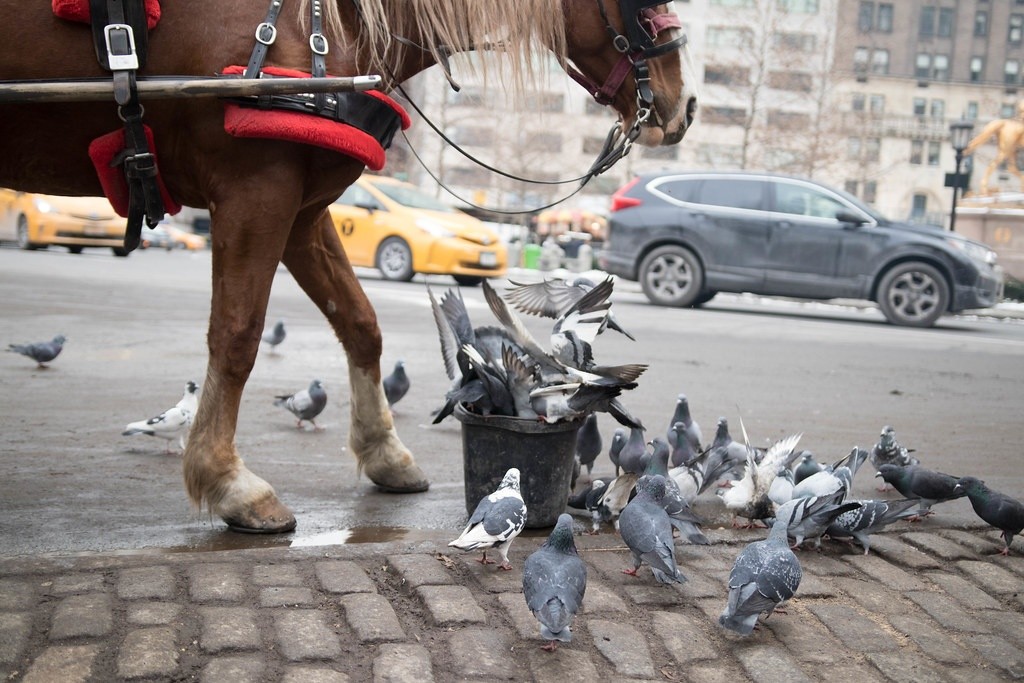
[594,167,1003,327]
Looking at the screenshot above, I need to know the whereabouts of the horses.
[0,0,699,535]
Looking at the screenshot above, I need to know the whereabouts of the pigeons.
[953,476,1024,557]
[718,521,803,635]
[520,512,588,653]
[273,380,327,431]
[424,267,936,556]
[260,321,285,349]
[618,474,689,589]
[874,463,986,523]
[120,381,200,458]
[447,468,528,570]
[4,334,68,370]
[382,361,411,407]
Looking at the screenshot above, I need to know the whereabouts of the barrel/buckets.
[450,400,584,532]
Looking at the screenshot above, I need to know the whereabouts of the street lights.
[945,110,976,233]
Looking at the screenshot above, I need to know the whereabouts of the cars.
[162,222,207,251]
[1,186,131,258]
[326,171,510,286]
[140,222,173,252]
